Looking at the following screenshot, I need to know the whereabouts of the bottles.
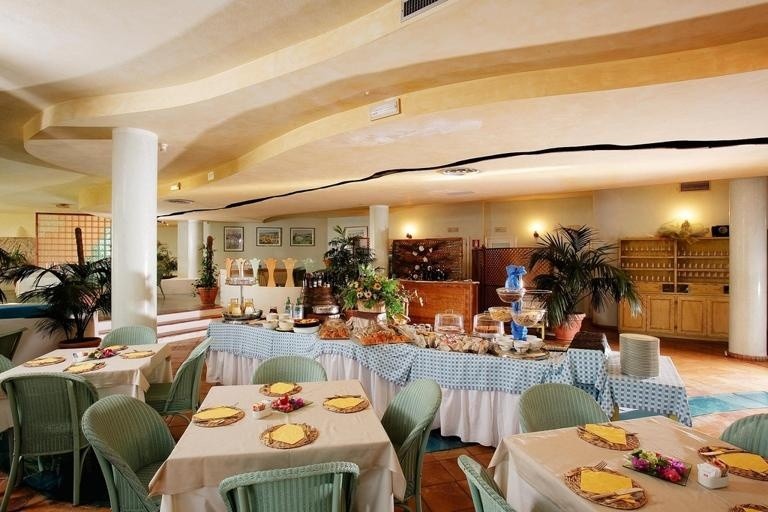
[225,298,257,315]
[472,310,504,337]
[434,309,465,333]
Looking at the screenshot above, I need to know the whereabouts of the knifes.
[591,487,644,500]
[700,449,748,455]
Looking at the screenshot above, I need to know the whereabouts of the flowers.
[339,269,404,316]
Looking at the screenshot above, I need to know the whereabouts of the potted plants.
[0,246,112,349]
[529,225,648,340]
[192,236,219,305]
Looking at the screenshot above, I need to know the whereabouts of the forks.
[562,460,607,478]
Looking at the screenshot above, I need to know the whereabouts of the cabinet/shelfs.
[618,235,729,343]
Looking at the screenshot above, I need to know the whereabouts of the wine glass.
[622,245,729,282]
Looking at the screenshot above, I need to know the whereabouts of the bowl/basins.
[263,312,294,332]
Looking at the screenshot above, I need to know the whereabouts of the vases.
[357,299,382,313]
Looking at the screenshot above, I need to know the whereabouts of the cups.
[498,333,546,353]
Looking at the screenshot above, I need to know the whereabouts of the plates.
[619,333,661,379]
[622,452,693,487]
[268,398,313,414]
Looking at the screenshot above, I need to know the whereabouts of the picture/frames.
[256,227,282,247]
[290,227,316,246]
[224,226,244,252]
